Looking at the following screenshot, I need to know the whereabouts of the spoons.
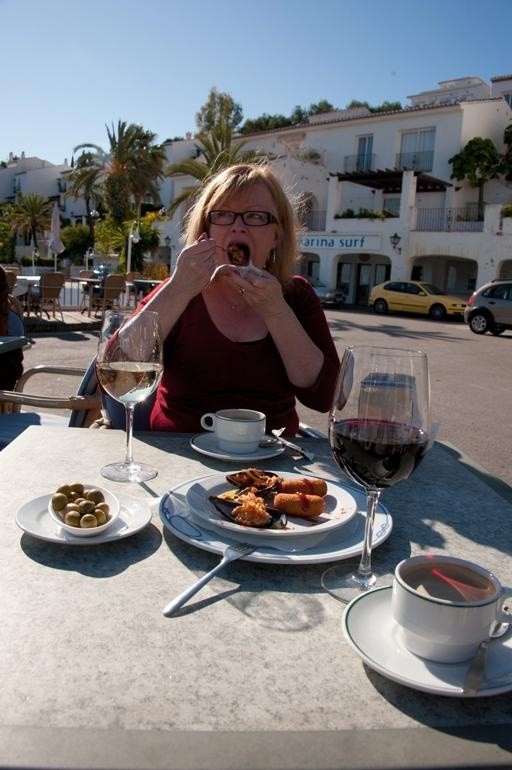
[461,611,509,696]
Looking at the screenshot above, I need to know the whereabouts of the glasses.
[205,210,279,227]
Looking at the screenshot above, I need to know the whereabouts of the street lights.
[125,219,139,307]
[31,246,95,273]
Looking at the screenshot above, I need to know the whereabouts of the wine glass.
[96,310,164,482]
[320,346,430,604]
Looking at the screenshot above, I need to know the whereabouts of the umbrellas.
[48,201,66,273]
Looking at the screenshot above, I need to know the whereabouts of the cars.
[306,275,346,309]
[368,280,512,334]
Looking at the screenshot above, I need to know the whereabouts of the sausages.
[274,491,327,518]
[277,478,328,497]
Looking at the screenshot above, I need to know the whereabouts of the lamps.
[389,232,401,255]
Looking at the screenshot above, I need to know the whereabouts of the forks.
[162,542,257,618]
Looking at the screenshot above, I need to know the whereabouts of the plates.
[189,432,287,461]
[15,484,151,545]
[341,585,512,698]
[161,471,394,566]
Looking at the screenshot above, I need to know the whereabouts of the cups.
[199,409,268,452]
[390,554,512,664]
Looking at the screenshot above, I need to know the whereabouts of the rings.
[237,287,247,297]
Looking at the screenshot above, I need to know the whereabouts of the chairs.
[0,309,124,449]
[0,266,143,324]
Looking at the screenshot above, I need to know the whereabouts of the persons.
[0,263,23,415]
[94,162,342,440]
